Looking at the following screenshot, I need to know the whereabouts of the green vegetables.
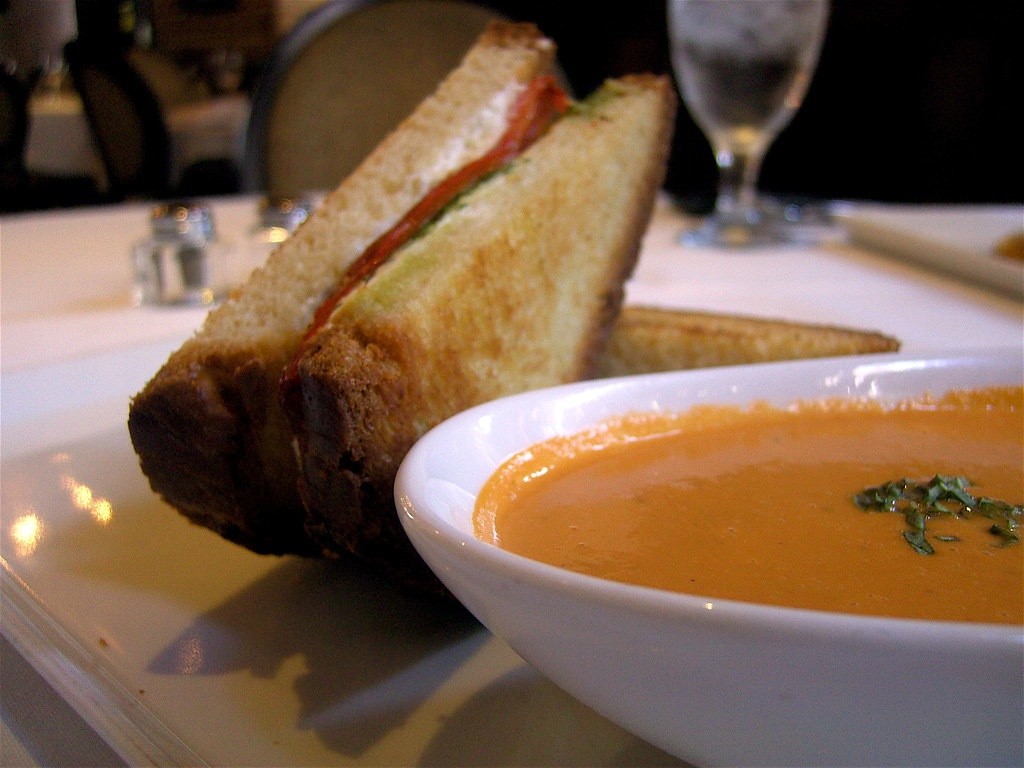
[848,475,1024,558]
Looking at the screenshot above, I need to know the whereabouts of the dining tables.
[0,201,1024,768]
[26,89,252,187]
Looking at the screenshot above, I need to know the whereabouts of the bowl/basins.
[394,343,1024,768]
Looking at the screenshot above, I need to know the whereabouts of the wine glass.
[667,0,852,254]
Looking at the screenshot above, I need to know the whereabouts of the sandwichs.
[127,22,674,560]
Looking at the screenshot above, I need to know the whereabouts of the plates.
[1,416,673,768]
[830,203,1024,301]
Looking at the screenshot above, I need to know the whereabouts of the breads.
[597,301,905,373]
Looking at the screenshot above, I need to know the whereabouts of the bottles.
[128,195,318,309]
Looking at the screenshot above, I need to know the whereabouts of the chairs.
[238,0,498,193]
[61,36,182,201]
